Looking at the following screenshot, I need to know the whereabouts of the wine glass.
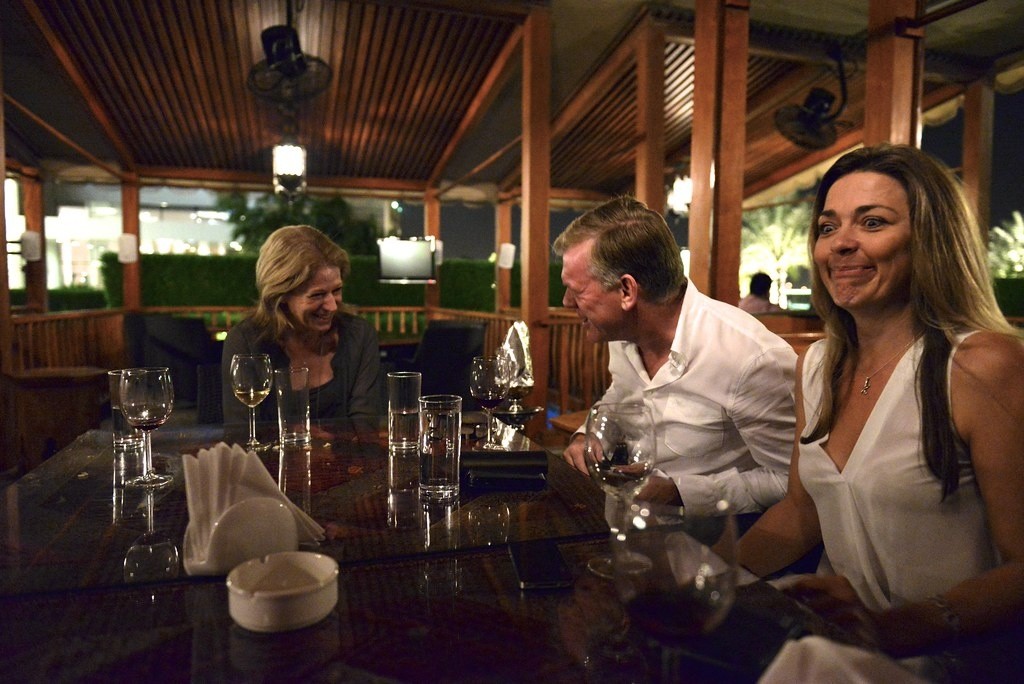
[123,486,180,582]
[120,366,176,488]
[470,355,510,452]
[584,401,658,580]
[229,353,273,450]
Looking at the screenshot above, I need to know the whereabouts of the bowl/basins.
[227,551,339,634]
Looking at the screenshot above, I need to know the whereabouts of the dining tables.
[0,412,664,599]
[0,531,888,683]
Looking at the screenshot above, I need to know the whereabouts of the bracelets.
[928,593,966,658]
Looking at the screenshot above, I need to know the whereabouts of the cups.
[108,369,148,451]
[278,445,311,507]
[275,366,312,445]
[388,371,421,453]
[418,394,462,504]
[113,452,148,525]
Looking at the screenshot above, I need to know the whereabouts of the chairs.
[118,313,487,427]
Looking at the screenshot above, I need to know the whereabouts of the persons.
[553,197,797,545]
[739,273,781,313]
[221,225,384,420]
[724,143,1024,684]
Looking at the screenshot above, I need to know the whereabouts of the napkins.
[762,637,925,683]
[177,446,327,559]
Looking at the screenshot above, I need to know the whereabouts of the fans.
[774,44,857,149]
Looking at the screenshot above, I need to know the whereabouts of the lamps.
[499,242,517,268]
[269,108,306,206]
[21,231,44,260]
[248,26,332,105]
[433,241,444,266]
[118,232,137,263]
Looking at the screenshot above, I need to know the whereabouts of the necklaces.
[856,330,920,395]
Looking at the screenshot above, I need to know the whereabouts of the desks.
[8,367,108,473]
